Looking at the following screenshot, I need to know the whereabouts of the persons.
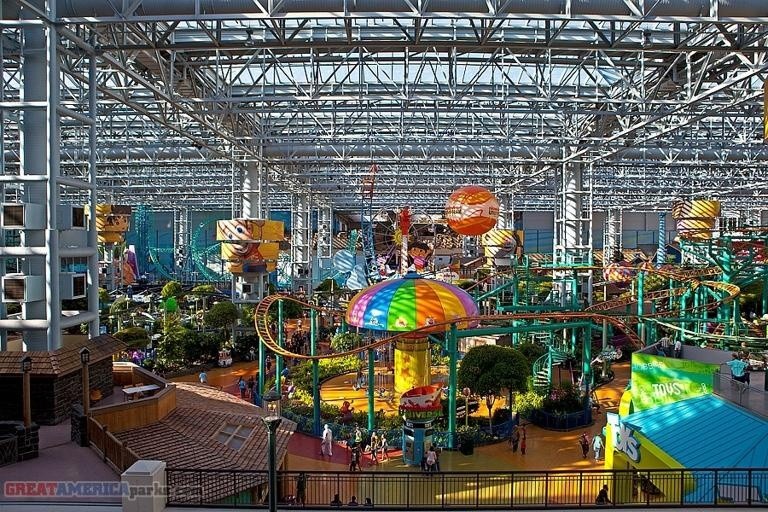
[198,316,768,507]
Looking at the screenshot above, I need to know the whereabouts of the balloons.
[446,187,499,237]
